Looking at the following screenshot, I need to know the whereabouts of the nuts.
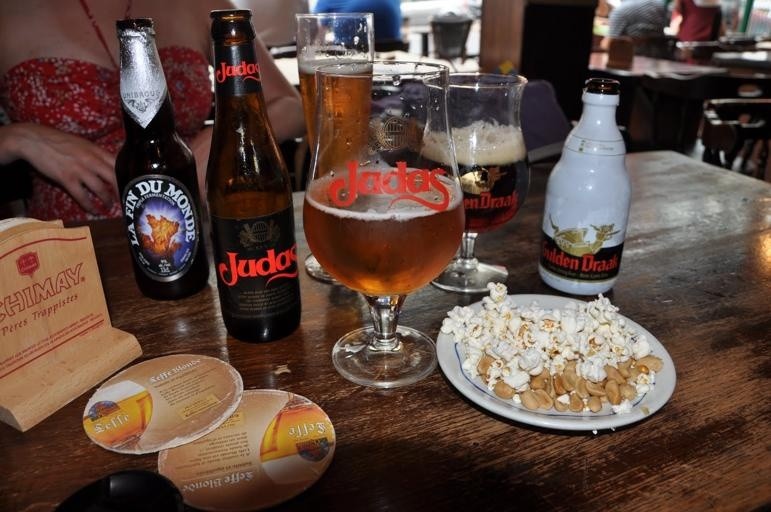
[477,356,664,413]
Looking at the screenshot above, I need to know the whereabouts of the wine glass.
[292,10,379,283]
[303,54,467,394]
[418,70,533,295]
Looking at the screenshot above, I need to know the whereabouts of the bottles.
[109,12,213,302]
[535,72,630,298]
[202,4,300,346]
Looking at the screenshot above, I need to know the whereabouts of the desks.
[586,46,729,160]
[712,42,770,69]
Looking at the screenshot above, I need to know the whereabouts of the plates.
[432,292,683,430]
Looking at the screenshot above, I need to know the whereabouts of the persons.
[244,0,319,59]
[1,1,308,220]
[664,0,723,62]
[599,0,671,57]
[313,0,402,49]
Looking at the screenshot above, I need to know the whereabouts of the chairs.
[701,97,771,179]
